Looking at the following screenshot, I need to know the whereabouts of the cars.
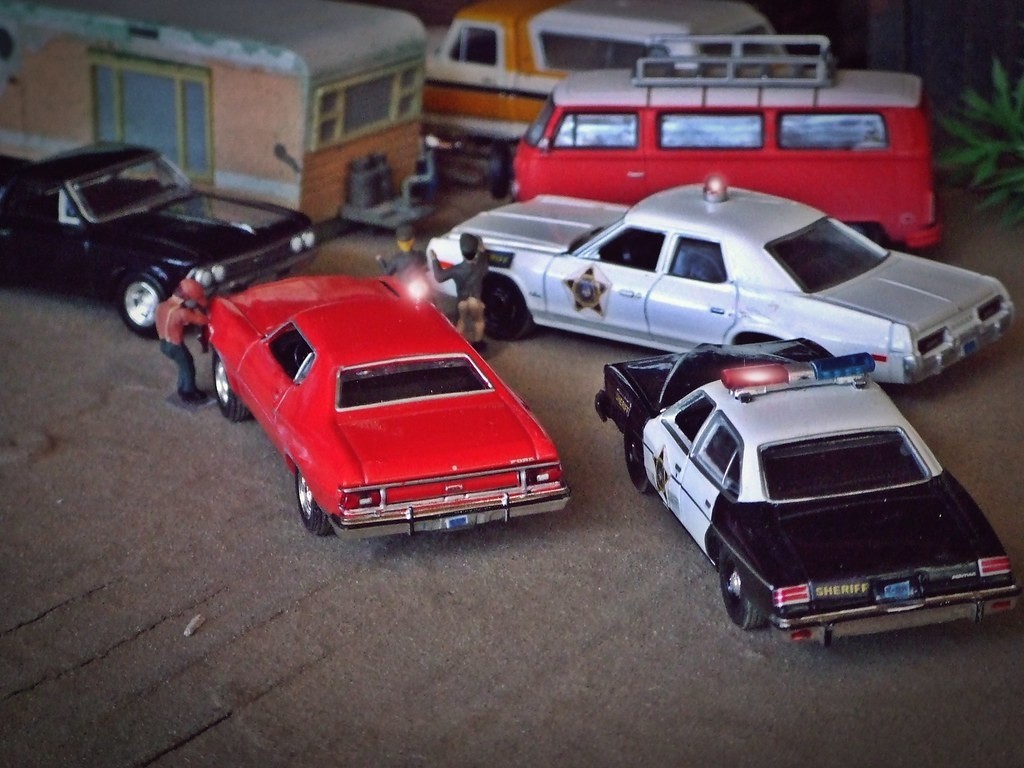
[421,169,1013,386]
[192,271,571,542]
[2,140,320,341]
[595,336,1024,650]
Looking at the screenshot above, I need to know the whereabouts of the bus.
[510,35,947,255]
[413,2,797,164]
